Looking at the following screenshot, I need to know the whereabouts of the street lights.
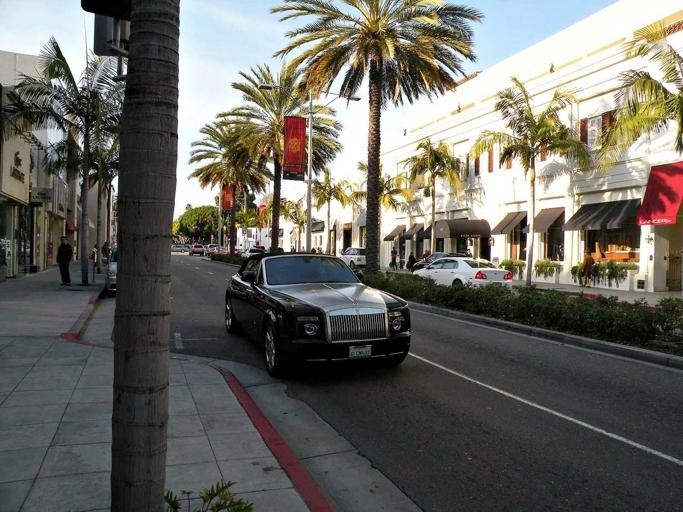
[257,84,362,254]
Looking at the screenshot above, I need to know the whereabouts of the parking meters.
[92,248,97,282]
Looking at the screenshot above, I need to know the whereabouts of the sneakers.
[61,282,71,285]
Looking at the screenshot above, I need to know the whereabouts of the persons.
[408,252,415,272]
[92,244,100,270]
[390,246,398,271]
[578,249,595,287]
[57,235,73,286]
[102,241,110,257]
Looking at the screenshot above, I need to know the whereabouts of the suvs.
[339,246,366,270]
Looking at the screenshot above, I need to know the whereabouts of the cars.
[411,251,513,292]
[102,245,117,292]
[171,244,265,259]
[223,251,412,381]
